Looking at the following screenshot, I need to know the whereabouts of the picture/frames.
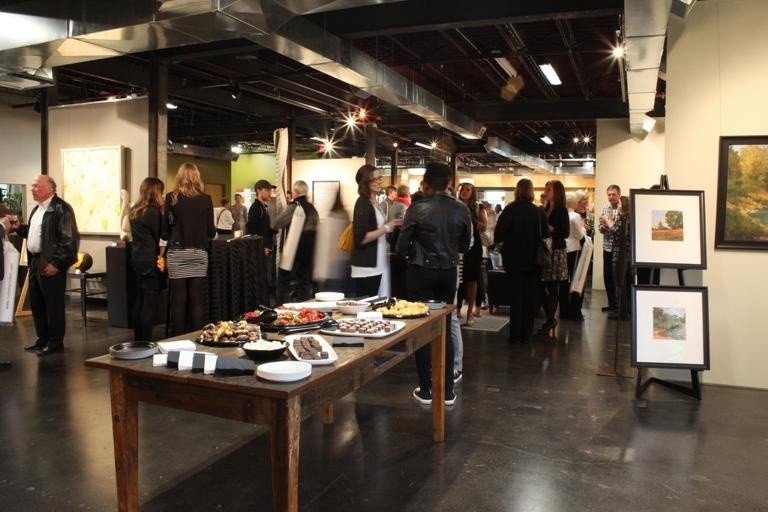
[632,284,709,371]
[714,134,768,251]
[628,186,707,270]
[60,144,127,236]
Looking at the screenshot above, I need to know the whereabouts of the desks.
[84,292,460,512]
[164,233,268,336]
[63,268,107,317]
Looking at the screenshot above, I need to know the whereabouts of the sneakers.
[413,387,432,404]
[445,391,458,405]
[453,369,463,383]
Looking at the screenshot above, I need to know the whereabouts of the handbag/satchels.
[536,236,554,270]
[337,222,353,253]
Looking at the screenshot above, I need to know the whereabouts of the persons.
[126,176,166,343]
[392,159,473,407]
[213,179,319,303]
[17,352,90,511]
[379,181,423,224]
[458,177,631,340]
[154,161,220,336]
[346,164,406,299]
[0,187,20,373]
[431,183,478,387]
[9,174,81,356]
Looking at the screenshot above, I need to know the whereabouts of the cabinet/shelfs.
[106,244,165,328]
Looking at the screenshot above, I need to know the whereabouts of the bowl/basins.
[335,301,371,314]
[240,339,290,360]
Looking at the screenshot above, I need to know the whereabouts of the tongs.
[371,296,395,310]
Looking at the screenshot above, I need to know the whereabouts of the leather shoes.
[38,342,64,357]
[24,340,47,350]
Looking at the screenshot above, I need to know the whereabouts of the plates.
[196,334,266,347]
[254,360,313,383]
[285,333,339,365]
[418,298,446,309]
[109,340,159,360]
[261,309,334,333]
[282,301,336,313]
[320,318,406,337]
[315,291,344,302]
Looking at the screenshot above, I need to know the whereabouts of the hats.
[255,180,277,189]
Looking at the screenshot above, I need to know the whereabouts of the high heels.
[538,320,557,337]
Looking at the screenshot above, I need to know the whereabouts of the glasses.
[370,176,384,182]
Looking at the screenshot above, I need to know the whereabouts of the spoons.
[246,310,277,324]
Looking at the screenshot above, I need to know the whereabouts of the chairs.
[485,244,509,313]
